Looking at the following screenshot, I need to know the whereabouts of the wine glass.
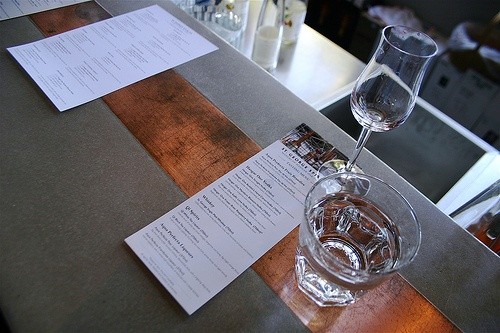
[316,25,438,197]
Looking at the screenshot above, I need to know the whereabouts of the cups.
[295,172,421,307]
[165,0,250,53]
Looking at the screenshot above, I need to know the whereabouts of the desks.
[0,0,500,333]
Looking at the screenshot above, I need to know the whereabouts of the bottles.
[251,0,284,73]
[277,0,308,46]
